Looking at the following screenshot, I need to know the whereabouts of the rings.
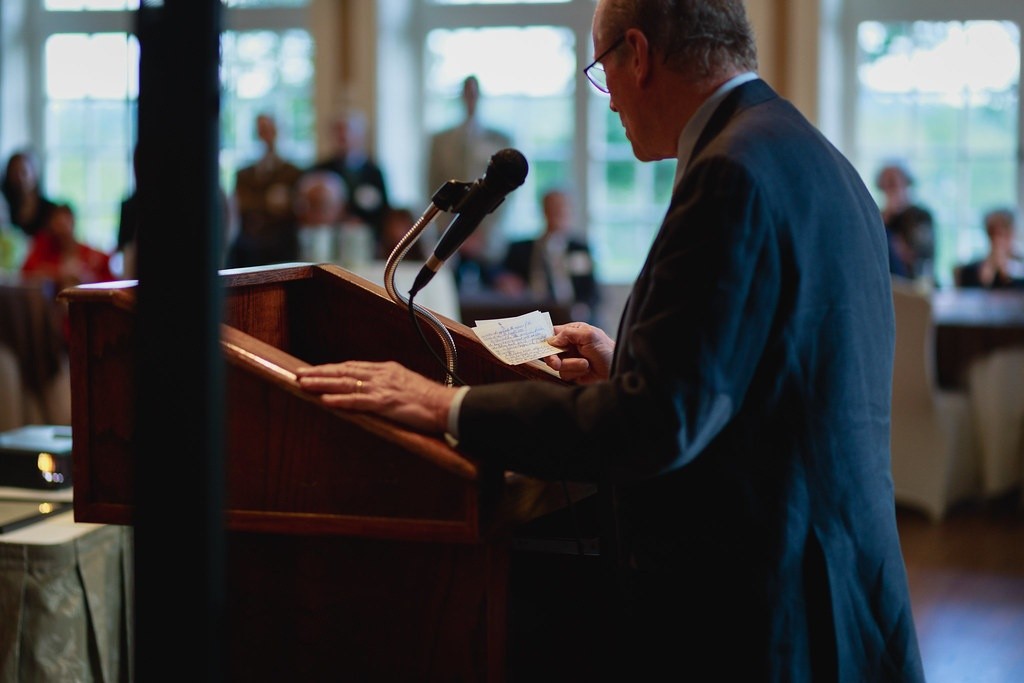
[357,379,364,391]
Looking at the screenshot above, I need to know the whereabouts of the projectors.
[0,424,74,491]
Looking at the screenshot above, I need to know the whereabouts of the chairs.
[888,282,980,523]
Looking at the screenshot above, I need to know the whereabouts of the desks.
[933,286,1024,391]
[0,482,135,683]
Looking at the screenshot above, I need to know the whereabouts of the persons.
[866,155,1024,293]
[228,100,425,268]
[296,1,929,682]
[1,147,120,424]
[450,187,603,329]
[428,73,514,264]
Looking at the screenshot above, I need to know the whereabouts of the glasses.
[583,37,626,93]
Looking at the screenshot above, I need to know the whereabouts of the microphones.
[410,148,528,296]
